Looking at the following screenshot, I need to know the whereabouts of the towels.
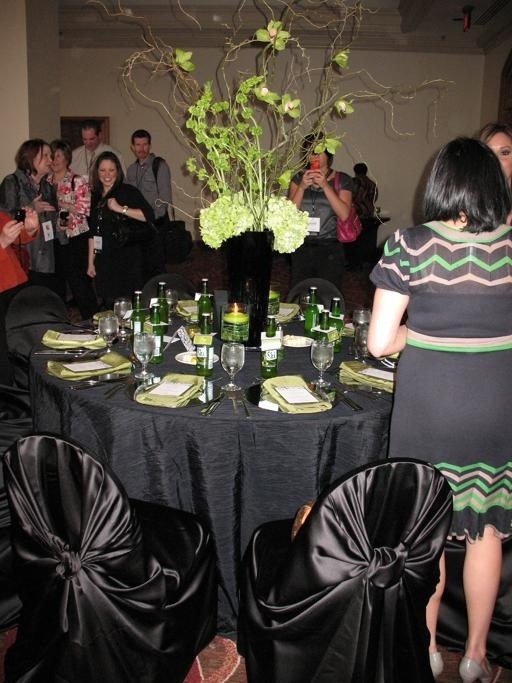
[45,351,133,381]
[175,299,214,323]
[92,309,150,329]
[261,374,332,415]
[339,360,396,394]
[136,373,206,408]
[41,329,108,349]
[342,321,355,339]
[267,302,300,324]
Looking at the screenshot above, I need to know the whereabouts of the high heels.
[429,652,445,680]
[459,657,497,683]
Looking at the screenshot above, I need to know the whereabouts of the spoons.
[237,392,250,419]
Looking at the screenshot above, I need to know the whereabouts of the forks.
[227,391,237,415]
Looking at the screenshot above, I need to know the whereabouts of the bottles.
[147,303,163,364]
[313,310,330,348]
[197,279,211,327]
[327,297,343,353]
[195,312,214,376]
[130,291,145,341]
[259,315,278,379]
[156,282,169,334]
[304,287,319,338]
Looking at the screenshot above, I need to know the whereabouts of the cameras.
[14,208,26,223]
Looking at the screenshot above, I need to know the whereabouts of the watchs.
[123,205,129,215]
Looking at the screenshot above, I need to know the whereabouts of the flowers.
[89,0,457,256]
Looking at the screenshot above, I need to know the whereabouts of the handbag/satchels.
[113,184,156,247]
[152,156,194,264]
[335,170,363,244]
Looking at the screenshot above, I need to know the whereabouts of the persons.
[366,135,512,683]
[0,137,57,287]
[122,129,173,235]
[46,140,100,319]
[472,120,511,225]
[68,118,126,191]
[0,204,41,329]
[86,151,157,311]
[351,162,379,258]
[285,130,357,291]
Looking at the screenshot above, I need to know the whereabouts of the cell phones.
[310,160,320,170]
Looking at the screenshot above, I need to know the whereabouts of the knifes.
[335,386,364,412]
[34,349,84,355]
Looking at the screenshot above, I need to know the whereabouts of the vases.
[226,231,275,347]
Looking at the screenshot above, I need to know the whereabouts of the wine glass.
[352,308,371,328]
[165,288,177,322]
[220,342,245,392]
[311,340,334,393]
[114,298,132,339]
[98,316,119,349]
[355,325,370,365]
[132,332,155,380]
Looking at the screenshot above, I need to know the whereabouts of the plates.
[174,350,219,366]
[284,335,313,348]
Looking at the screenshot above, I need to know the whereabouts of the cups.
[219,302,250,343]
[299,293,310,318]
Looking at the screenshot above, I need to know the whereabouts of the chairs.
[5,283,72,390]
[238,455,454,682]
[286,278,346,313]
[142,272,198,304]
[0,432,216,683]
